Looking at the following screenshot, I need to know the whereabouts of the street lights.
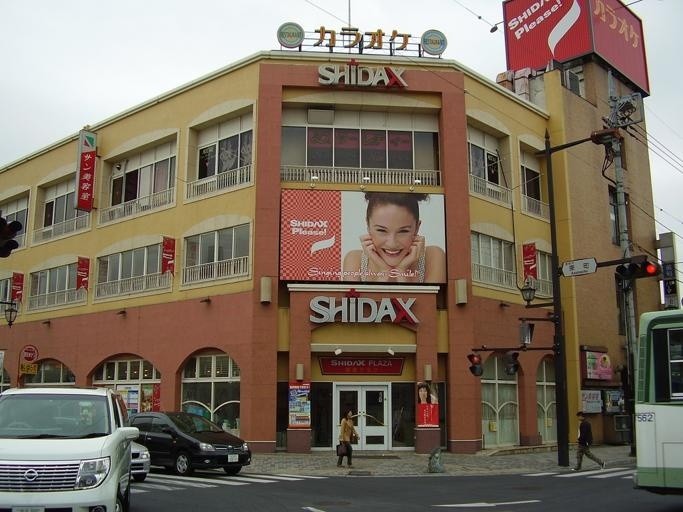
[0,299,18,329]
[519,125,624,466]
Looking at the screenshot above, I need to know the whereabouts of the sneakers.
[571,468,580,470]
[601,462,605,471]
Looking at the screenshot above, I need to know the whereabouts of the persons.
[417,382,437,405]
[568,412,606,473]
[336,406,360,469]
[341,192,446,283]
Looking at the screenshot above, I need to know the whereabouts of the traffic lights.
[503,351,519,375]
[0,218,22,258]
[615,261,661,278]
[467,352,482,377]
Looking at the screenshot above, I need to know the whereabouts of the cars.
[127,441,152,484]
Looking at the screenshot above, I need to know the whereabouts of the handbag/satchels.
[337,442,348,457]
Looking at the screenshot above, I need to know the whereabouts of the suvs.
[0,385,139,511]
[129,412,250,478]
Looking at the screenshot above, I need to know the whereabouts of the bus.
[632,309,682,495]
